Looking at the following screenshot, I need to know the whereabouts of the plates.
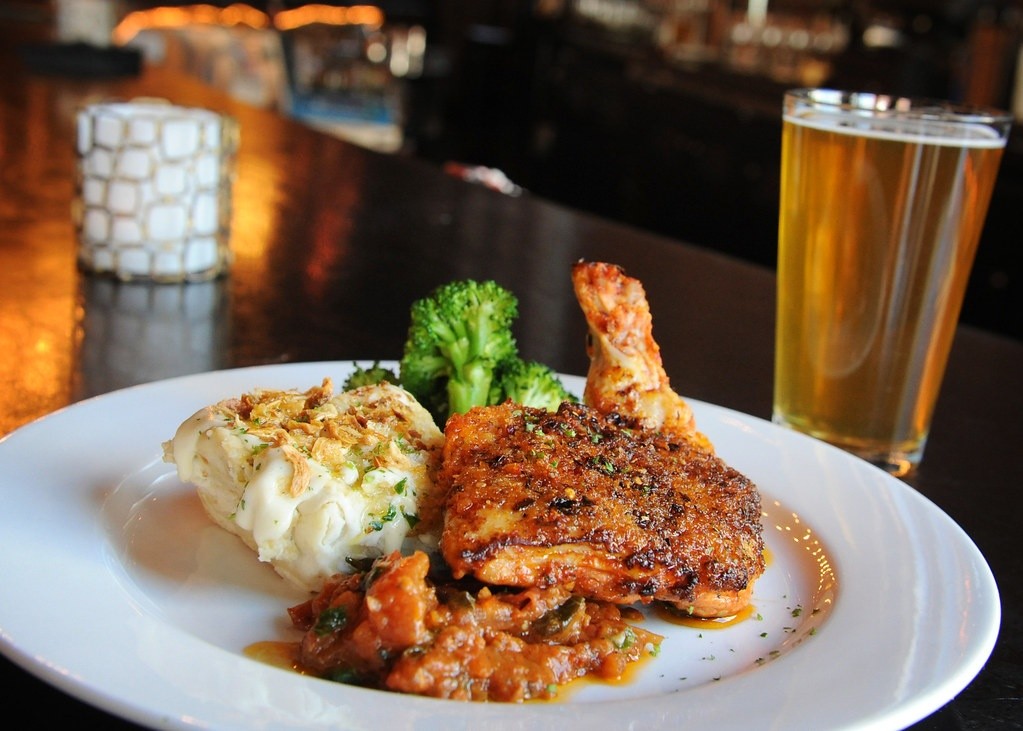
[1,361,1002,731]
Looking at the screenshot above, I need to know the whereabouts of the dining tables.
[0,61,1023,731]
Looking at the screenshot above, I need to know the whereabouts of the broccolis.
[340,276,579,430]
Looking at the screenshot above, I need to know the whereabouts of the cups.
[766,88,1015,480]
